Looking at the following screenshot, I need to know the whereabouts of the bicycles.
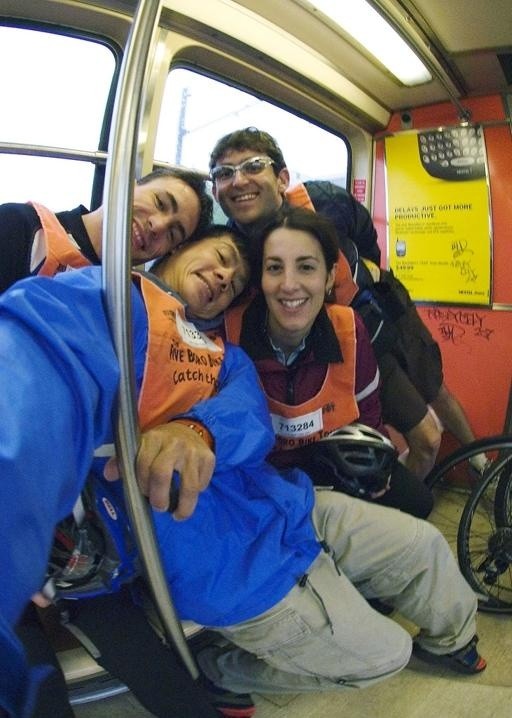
[453,447,512,608]
[424,435,512,615]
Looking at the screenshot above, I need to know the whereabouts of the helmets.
[38,470,147,603]
[310,421,399,498]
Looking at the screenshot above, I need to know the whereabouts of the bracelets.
[169,417,216,450]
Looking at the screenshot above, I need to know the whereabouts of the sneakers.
[412,632,487,675]
[194,655,258,718]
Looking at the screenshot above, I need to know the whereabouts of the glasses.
[209,156,276,179]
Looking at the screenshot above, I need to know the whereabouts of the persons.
[207,128,500,505]
[0,166,222,718]
[224,209,433,615]
[0,224,487,718]
